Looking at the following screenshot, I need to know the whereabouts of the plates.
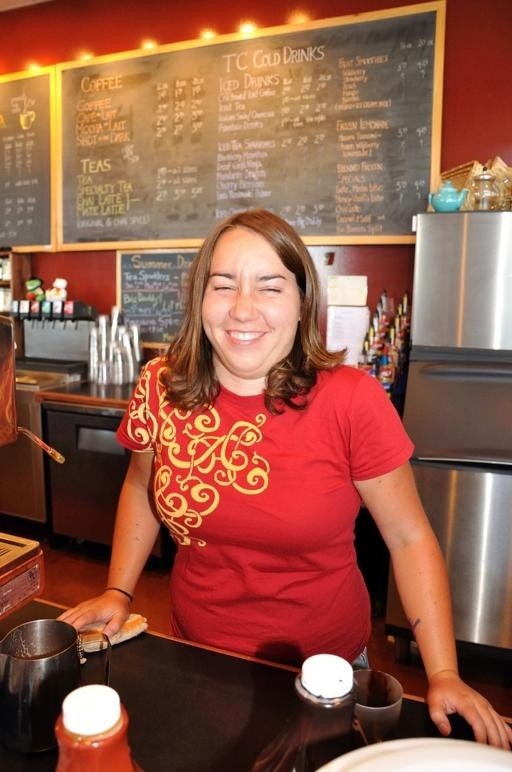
[312,737,511,772]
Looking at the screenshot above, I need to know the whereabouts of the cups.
[87,306,144,385]
[351,667,405,741]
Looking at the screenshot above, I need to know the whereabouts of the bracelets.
[102,586,133,602]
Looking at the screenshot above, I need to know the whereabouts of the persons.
[55,208,512,751]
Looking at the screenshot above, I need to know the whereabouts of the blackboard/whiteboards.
[54,0,448,254]
[0,65,56,253]
[115,248,201,350]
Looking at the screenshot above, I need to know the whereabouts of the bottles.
[465,173,504,212]
[50,683,143,772]
[232,654,371,771]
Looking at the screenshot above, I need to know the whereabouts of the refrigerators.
[382,213,511,657]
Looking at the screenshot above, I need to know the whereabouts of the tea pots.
[0,619,113,752]
[428,179,470,213]
